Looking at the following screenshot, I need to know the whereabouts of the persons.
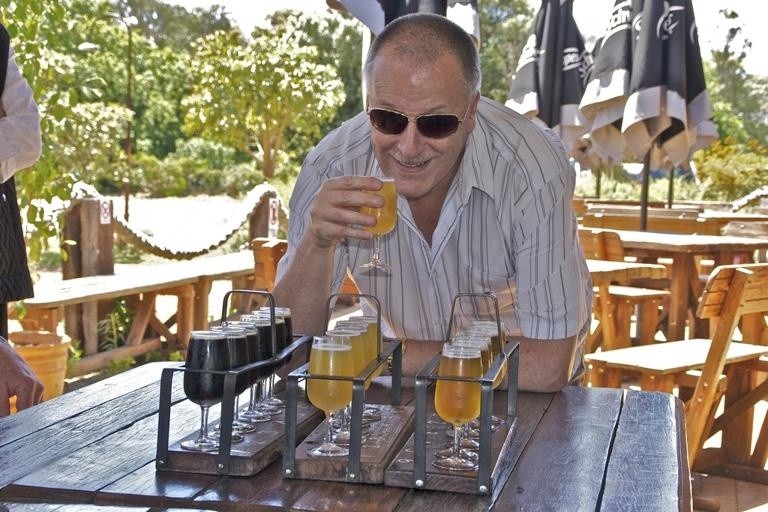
[256,11,593,396]
[1,339,49,417]
[0,24,44,350]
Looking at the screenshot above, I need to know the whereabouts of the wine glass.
[359,176,398,268]
[432,319,505,472]
[180,307,293,454]
[304,315,381,459]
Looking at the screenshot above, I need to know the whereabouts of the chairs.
[583,262,768,512]
[571,198,768,350]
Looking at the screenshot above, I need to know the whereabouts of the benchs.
[23,251,256,379]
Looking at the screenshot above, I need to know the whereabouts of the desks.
[0,360,694,512]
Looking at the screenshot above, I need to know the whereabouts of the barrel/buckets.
[9,333,72,413]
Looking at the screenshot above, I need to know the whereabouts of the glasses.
[363,91,474,141]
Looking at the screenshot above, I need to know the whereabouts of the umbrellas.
[502,0,596,164]
[576,0,721,230]
[326,0,484,113]
